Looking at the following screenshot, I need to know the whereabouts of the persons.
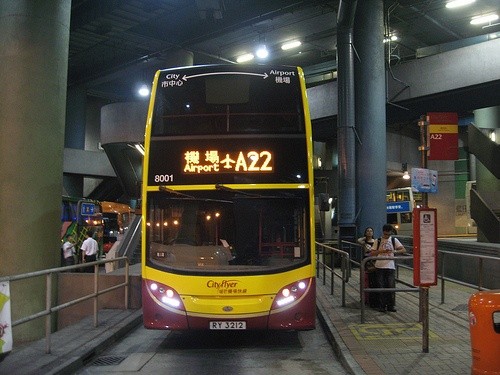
[63,234,78,273]
[423,214,431,223]
[370,224,406,312]
[81,231,99,273]
[103,232,115,254]
[357,227,375,262]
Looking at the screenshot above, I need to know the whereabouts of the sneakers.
[387,305,396,312]
[379,305,385,312]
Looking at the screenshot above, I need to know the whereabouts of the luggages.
[367,259,377,306]
[363,260,371,304]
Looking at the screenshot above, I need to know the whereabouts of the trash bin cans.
[468,289,500,375]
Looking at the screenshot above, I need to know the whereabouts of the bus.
[61,196,102,270]
[387,187,428,237]
[140,63,317,335]
[100,202,139,252]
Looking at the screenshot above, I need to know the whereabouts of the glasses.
[385,234,390,235]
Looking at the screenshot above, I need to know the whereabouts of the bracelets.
[394,250,397,254]
